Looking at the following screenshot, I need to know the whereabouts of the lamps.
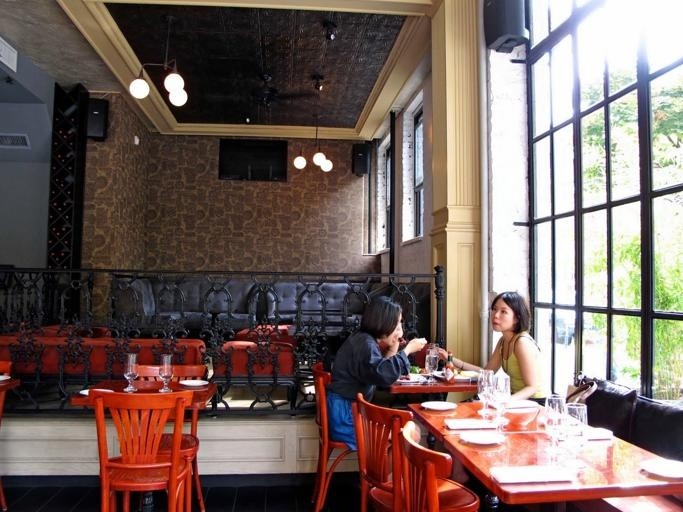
[129,15,189,107]
[293,113,334,172]
[314,82,323,91]
[325,30,335,40]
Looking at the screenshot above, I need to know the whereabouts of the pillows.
[584,377,640,441]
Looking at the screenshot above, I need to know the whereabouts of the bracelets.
[461,361,464,370]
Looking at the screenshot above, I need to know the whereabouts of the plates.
[395,373,427,385]
[79,389,114,397]
[640,458,683,480]
[444,418,495,430]
[0,375,11,384]
[458,431,506,445]
[490,464,572,484]
[178,380,208,388]
[421,401,457,412]
[435,370,481,383]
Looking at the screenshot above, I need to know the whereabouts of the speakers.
[87,97,109,141]
[483,0,531,54]
[351,143,372,176]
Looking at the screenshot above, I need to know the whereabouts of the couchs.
[217,339,295,380]
[0,322,204,379]
[113,277,431,336]
[234,323,288,345]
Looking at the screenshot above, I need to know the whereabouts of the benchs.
[576,371,682,512]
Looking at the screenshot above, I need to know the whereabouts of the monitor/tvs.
[218,139,288,182]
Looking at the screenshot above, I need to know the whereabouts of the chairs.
[0,360,13,375]
[88,389,194,512]
[118,362,206,512]
[310,361,357,512]
[351,391,479,512]
[397,419,453,512]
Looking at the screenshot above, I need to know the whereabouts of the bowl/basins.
[500,400,539,427]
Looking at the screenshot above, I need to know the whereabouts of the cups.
[428,343,439,361]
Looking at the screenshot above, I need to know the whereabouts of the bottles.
[444,351,454,385]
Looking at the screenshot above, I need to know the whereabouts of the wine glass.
[544,394,565,460]
[424,348,437,383]
[563,404,589,465]
[475,370,493,422]
[490,375,510,433]
[158,354,174,393]
[121,353,139,393]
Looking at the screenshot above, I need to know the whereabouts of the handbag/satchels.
[564,385,586,404]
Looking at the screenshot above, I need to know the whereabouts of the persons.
[427,291,553,486]
[319,296,428,491]
